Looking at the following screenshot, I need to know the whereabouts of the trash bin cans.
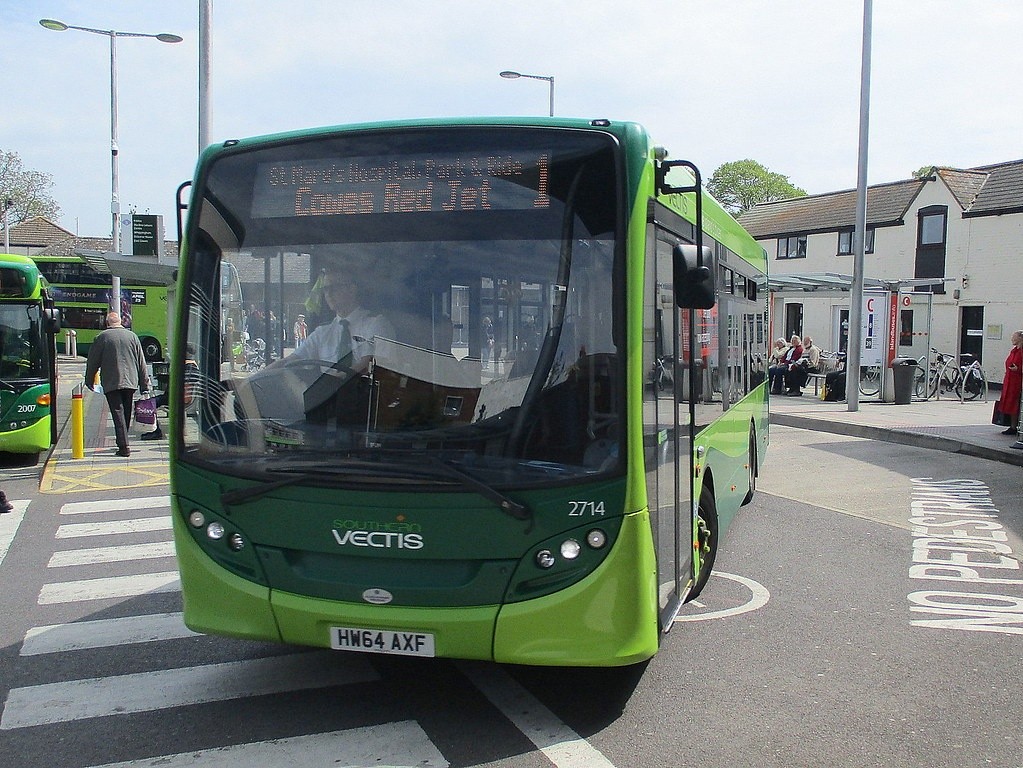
[890,357,920,404]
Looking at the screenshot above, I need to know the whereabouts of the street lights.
[40,19,184,323]
[500,72,554,116]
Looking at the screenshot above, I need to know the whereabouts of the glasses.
[323,282,354,292]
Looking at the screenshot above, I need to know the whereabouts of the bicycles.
[916,347,984,400]
[859,355,925,396]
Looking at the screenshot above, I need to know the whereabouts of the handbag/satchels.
[991,400,1011,427]
[965,370,982,396]
[134,393,156,424]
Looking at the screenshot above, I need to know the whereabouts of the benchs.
[160,381,199,424]
[807,358,839,396]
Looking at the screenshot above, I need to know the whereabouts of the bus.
[168,119,770,666]
[0,254,60,453]
[28,256,249,369]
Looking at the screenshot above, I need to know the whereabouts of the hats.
[298,315,305,318]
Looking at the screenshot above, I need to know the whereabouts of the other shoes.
[1002,427,1017,436]
[783,388,802,396]
[770,389,782,395]
[116,451,130,457]
[141,430,167,440]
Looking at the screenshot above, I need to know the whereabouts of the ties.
[337,320,353,374]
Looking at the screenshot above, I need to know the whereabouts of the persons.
[769,335,804,396]
[1009,374,1023,450]
[294,314,308,348]
[234,266,400,455]
[786,336,820,397]
[225,317,240,363]
[767,336,788,395]
[140,341,201,441]
[85,311,152,457]
[480,316,497,370]
[246,304,277,356]
[997,330,1023,435]
[523,315,540,350]
